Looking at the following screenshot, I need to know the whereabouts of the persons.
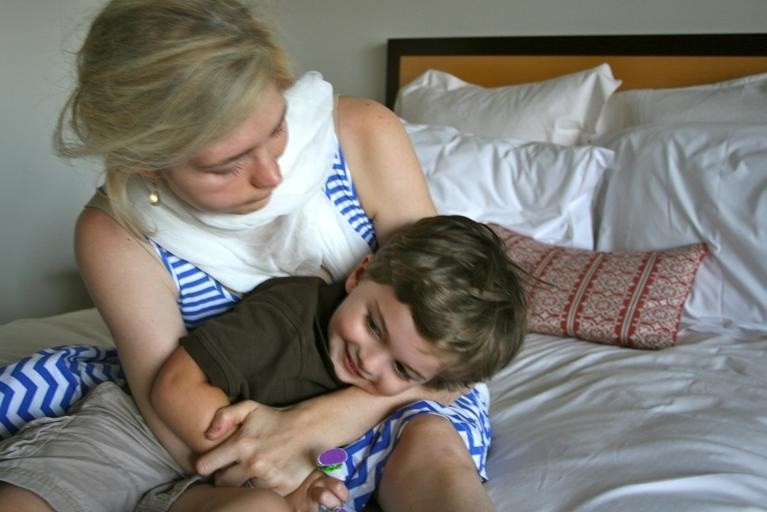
[0,214,531,512]
[53,0,501,512]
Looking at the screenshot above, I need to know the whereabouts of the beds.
[0,32,767,512]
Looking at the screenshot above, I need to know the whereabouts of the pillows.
[397,66,619,148]
[596,112,767,332]
[396,114,596,250]
[473,215,709,348]
[605,73,767,144]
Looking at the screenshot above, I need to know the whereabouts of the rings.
[242,480,254,489]
